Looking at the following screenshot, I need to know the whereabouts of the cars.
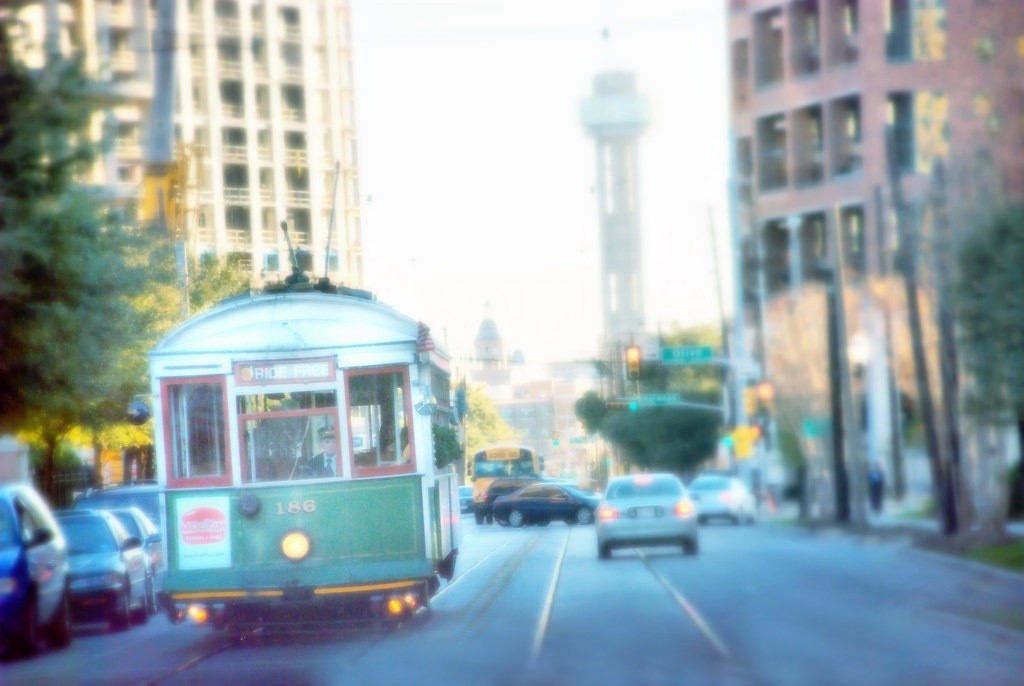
[48,507,161,634]
[686,476,757,526]
[483,475,601,528]
[594,474,699,561]
[459,486,475,514]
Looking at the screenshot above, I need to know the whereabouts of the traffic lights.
[604,401,638,411]
[626,345,642,380]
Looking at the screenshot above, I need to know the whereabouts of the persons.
[867,460,884,514]
[367,419,411,465]
[308,426,337,477]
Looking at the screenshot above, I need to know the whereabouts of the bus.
[125,160,462,642]
[467,444,545,525]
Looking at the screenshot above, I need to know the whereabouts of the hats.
[318,427,336,439]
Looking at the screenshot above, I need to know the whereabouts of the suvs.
[0,482,72,659]
[73,477,160,532]
